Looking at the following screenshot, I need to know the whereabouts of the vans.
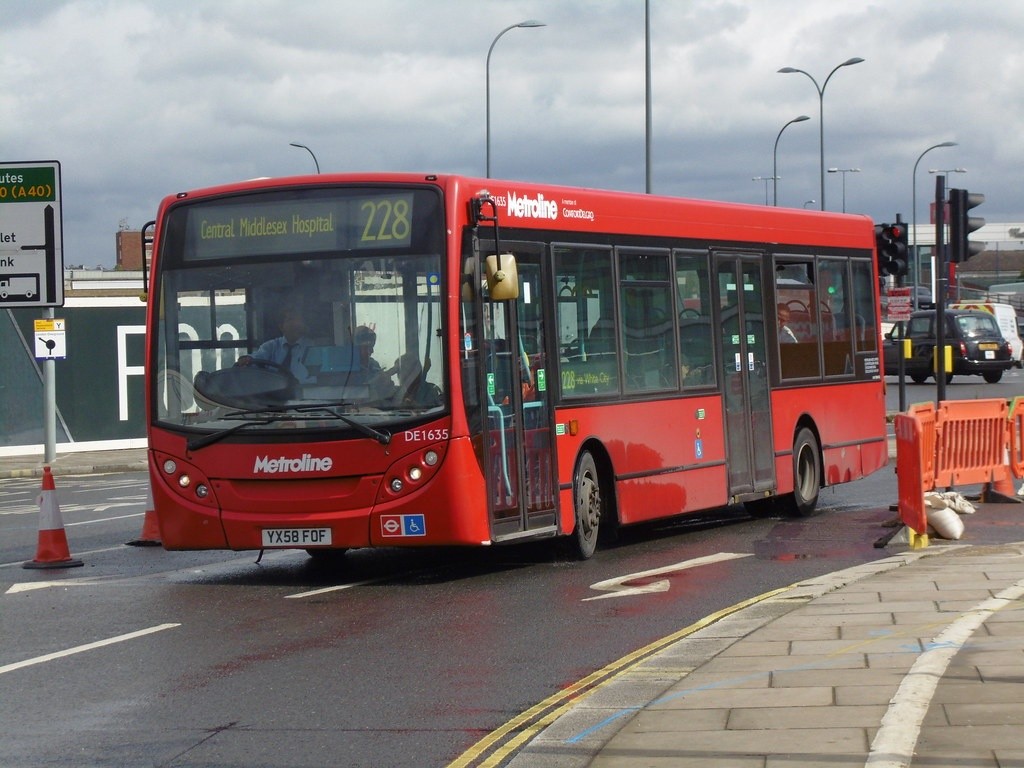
[949,304,1024,369]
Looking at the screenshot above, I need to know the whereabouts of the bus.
[130,172,887,572]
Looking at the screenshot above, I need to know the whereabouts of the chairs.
[484,300,864,408]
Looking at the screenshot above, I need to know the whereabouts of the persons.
[375,354,441,402]
[238,313,321,385]
[338,326,384,385]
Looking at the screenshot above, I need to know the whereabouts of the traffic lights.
[872,223,890,277]
[950,189,991,264]
[882,223,910,277]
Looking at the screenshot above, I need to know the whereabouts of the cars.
[907,286,934,308]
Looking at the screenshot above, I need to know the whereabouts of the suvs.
[882,309,1016,386]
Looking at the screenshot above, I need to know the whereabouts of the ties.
[279,343,299,370]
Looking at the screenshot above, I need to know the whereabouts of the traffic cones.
[19,464,89,571]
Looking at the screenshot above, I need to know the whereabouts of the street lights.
[752,176,780,204]
[290,142,321,176]
[776,56,865,211]
[482,21,545,174]
[927,167,967,200]
[826,167,860,216]
[772,115,810,205]
[910,141,960,312]
